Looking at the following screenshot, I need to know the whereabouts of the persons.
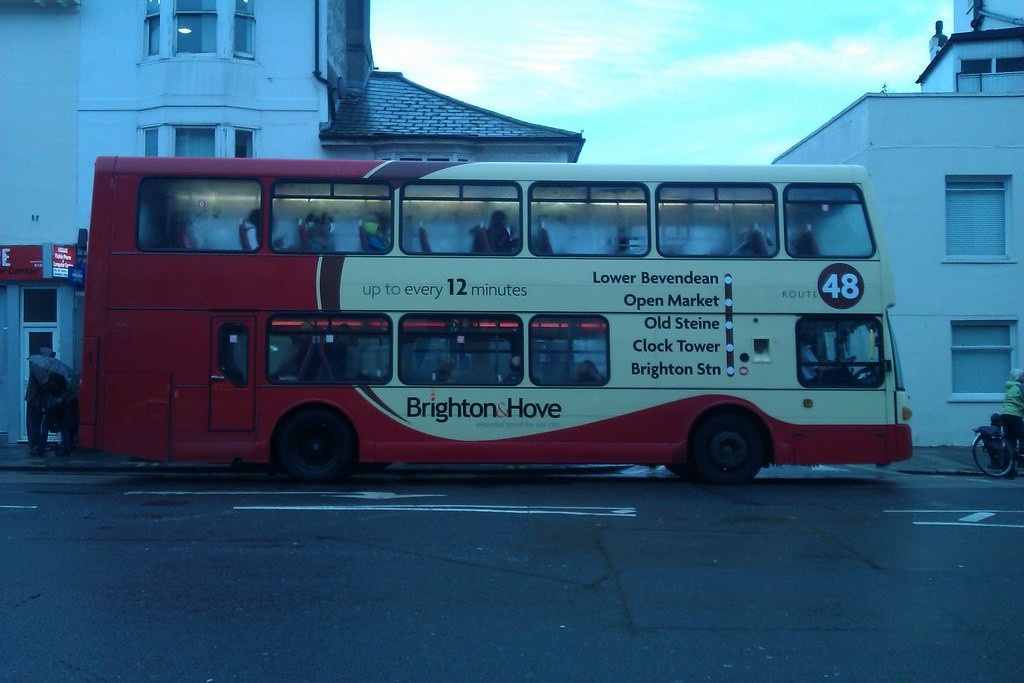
[488,211,513,253]
[24,347,73,455]
[800,327,853,385]
[243,209,261,252]
[303,210,335,251]
[1002,368,1024,477]
[360,209,390,251]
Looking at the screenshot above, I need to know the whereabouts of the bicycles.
[970,413,1024,478]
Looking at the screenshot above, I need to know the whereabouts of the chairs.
[474,226,492,254]
[240,224,255,253]
[358,225,372,253]
[179,209,199,250]
[419,227,435,252]
[542,228,555,254]
[298,226,316,252]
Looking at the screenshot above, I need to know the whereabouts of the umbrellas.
[26,354,73,382]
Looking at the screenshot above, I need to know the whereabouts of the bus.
[76,154,913,487]
